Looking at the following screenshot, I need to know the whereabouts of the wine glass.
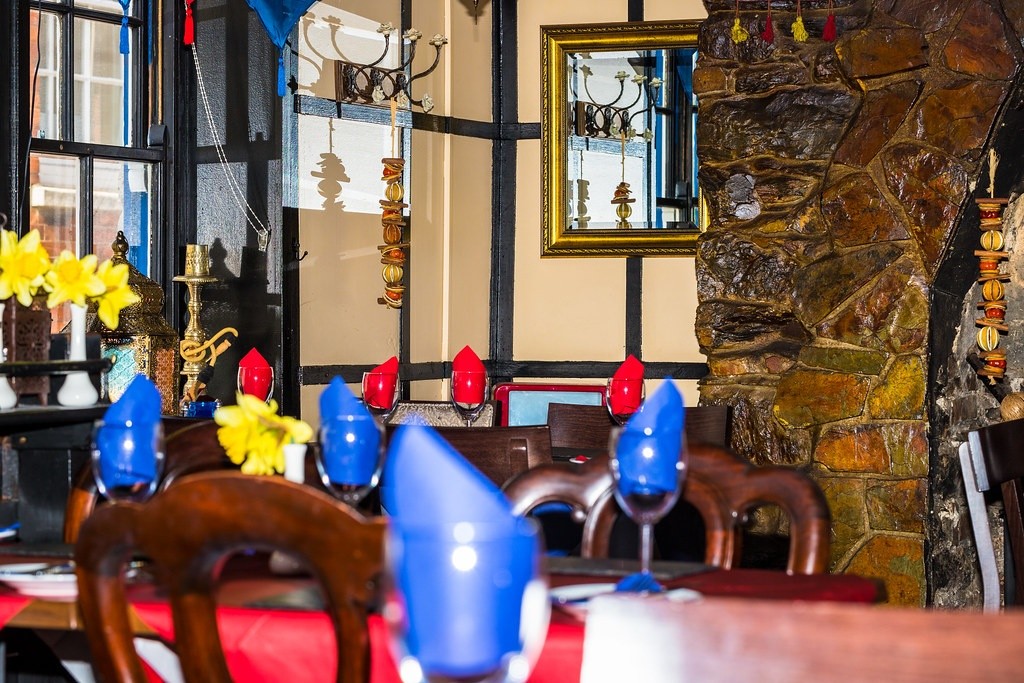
[609,421,687,598]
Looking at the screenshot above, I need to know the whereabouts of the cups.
[91,420,167,505]
[450,367,490,427]
[606,377,645,427]
[379,510,548,683]
[237,364,275,407]
[361,372,400,425]
[315,415,388,506]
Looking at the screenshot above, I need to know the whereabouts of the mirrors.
[536,18,712,258]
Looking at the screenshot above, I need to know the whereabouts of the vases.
[3,297,65,404]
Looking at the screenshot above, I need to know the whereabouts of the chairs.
[499,445,836,581]
[66,470,391,683]
[957,418,1023,614]
[579,592,1024,683]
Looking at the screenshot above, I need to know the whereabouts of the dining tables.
[0,531,896,655]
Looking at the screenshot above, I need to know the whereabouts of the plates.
[549,579,702,625]
[1,559,89,600]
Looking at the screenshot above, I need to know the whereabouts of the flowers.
[0,229,139,335]
[213,391,310,477]
